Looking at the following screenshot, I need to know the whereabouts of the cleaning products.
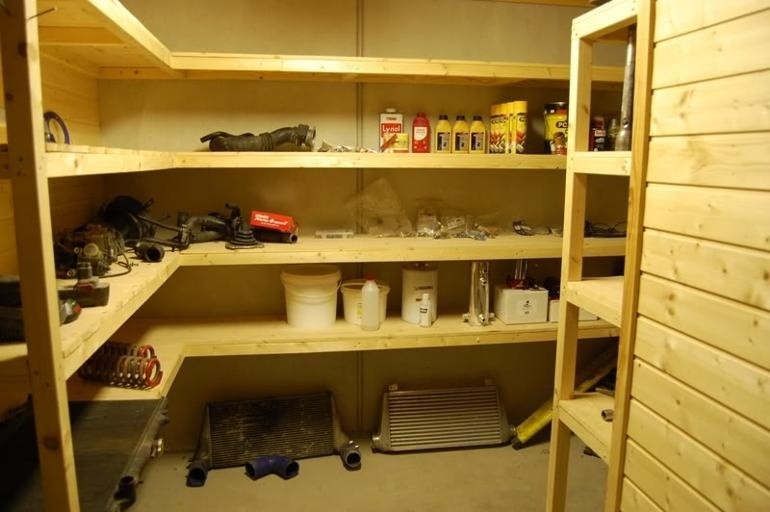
[411,99,529,154]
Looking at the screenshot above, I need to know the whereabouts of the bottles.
[418,294,432,328]
[412,100,528,153]
[589,115,619,151]
[361,274,380,332]
[379,108,403,153]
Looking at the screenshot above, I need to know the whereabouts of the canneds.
[543,102,569,155]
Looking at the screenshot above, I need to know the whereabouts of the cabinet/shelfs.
[0,0,649,511]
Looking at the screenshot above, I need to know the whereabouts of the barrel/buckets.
[339,277,391,327]
[279,264,344,330]
